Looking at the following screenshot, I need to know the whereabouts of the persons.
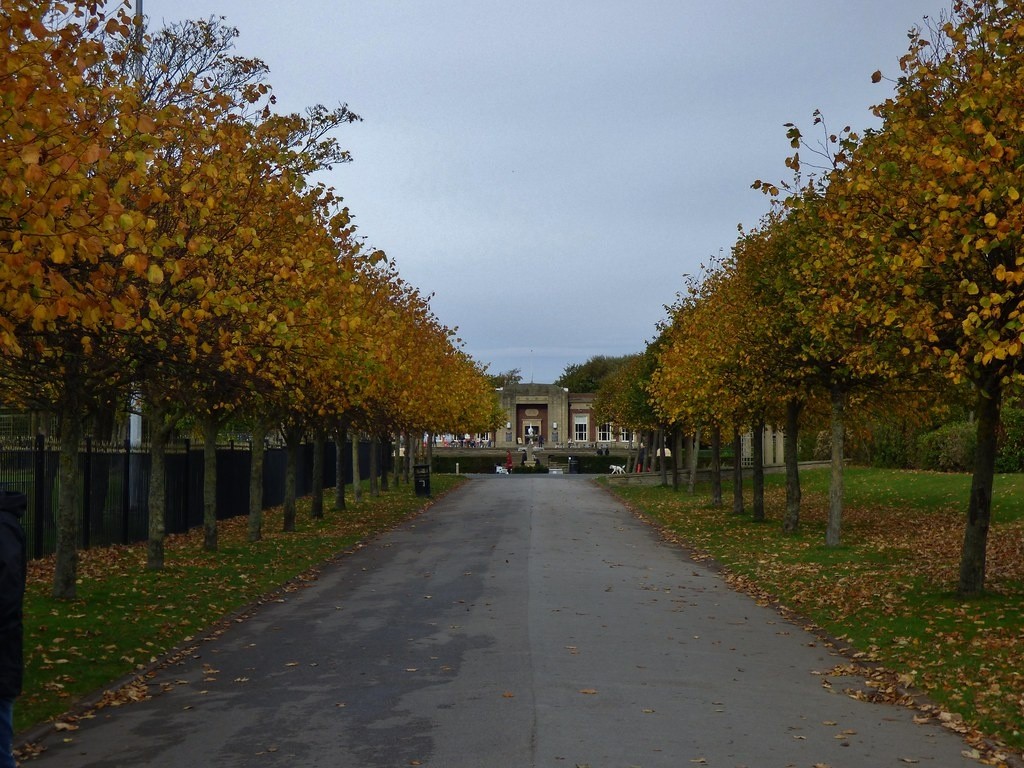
[539,435,544,448]
[503,449,513,473]
[423,436,493,448]
[519,448,527,465]
[596,448,610,456]
[566,436,573,449]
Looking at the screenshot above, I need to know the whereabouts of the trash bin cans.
[461,441,463,446]
[568,455,579,474]
[414,465,431,498]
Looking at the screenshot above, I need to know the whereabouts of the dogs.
[610,465,626,475]
